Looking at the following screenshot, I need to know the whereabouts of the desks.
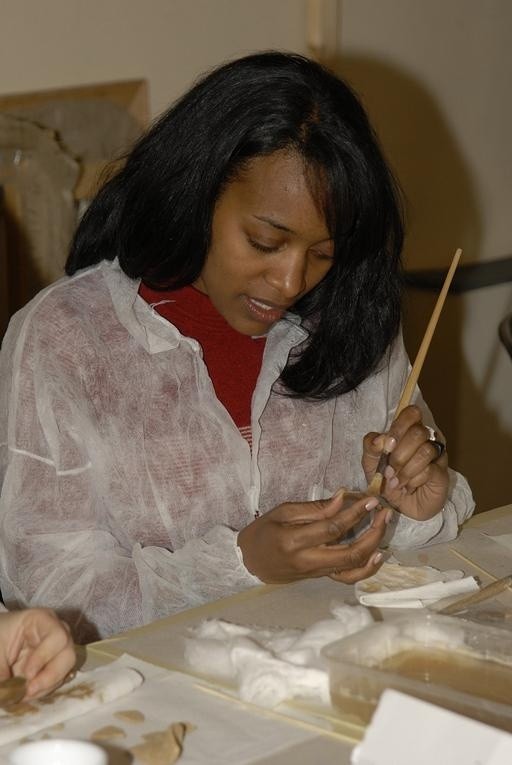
[0,504,512,765]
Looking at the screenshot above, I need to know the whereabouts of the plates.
[12,739,110,765]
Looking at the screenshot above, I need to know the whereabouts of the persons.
[1,602,77,698]
[1,48,476,646]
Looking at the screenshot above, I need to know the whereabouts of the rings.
[424,425,438,441]
[433,442,446,456]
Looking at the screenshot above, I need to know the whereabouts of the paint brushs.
[364,248,463,496]
